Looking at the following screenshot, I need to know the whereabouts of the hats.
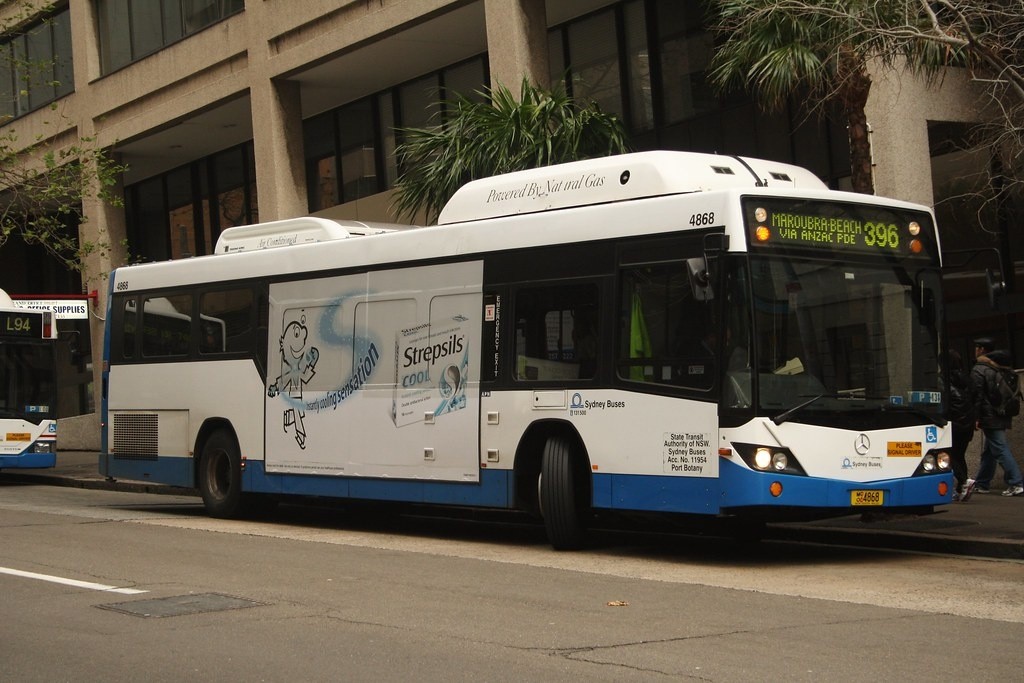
[975,338,993,347]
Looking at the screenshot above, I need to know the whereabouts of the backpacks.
[975,361,1023,418]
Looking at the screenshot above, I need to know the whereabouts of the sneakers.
[961,478,975,502]
[953,490,960,501]
[973,487,988,494]
[1001,485,1024,496]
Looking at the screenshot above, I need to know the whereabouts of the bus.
[96,147,1012,555]
[0,287,83,477]
[122,295,228,358]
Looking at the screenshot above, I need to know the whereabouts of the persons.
[679,323,733,397]
[198,319,222,353]
[968,334,1024,497]
[721,325,770,420]
[944,339,978,503]
